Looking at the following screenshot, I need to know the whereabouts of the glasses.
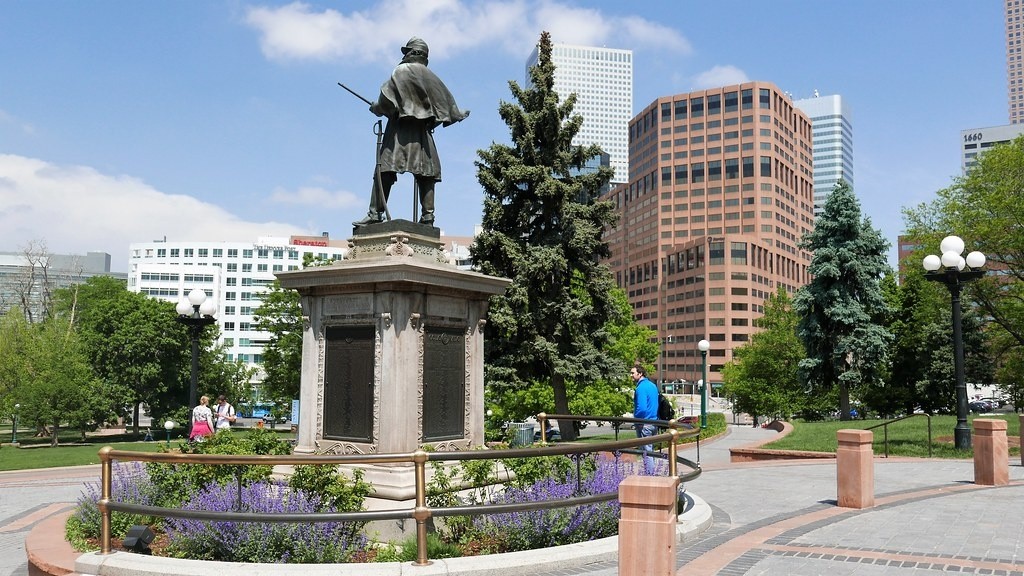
[219,401,223,404]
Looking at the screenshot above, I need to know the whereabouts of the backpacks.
[217,404,232,426]
[657,391,675,433]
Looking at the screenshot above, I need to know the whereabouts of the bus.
[236,403,288,418]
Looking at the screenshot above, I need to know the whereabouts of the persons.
[189,395,236,440]
[355,36,470,225]
[630,366,659,452]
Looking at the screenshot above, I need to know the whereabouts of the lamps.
[165,421,175,431]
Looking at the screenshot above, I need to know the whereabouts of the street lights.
[923,235,987,450]
[698,340,710,429]
[175,290,218,442]
[253,386,258,402]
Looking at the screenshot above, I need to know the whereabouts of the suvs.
[263,414,287,424]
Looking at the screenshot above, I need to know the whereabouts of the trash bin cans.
[505,421,535,447]
[271,420,275,428]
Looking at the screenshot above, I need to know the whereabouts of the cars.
[535,429,562,441]
[614,422,635,430]
[969,396,1009,414]
[677,416,698,424]
